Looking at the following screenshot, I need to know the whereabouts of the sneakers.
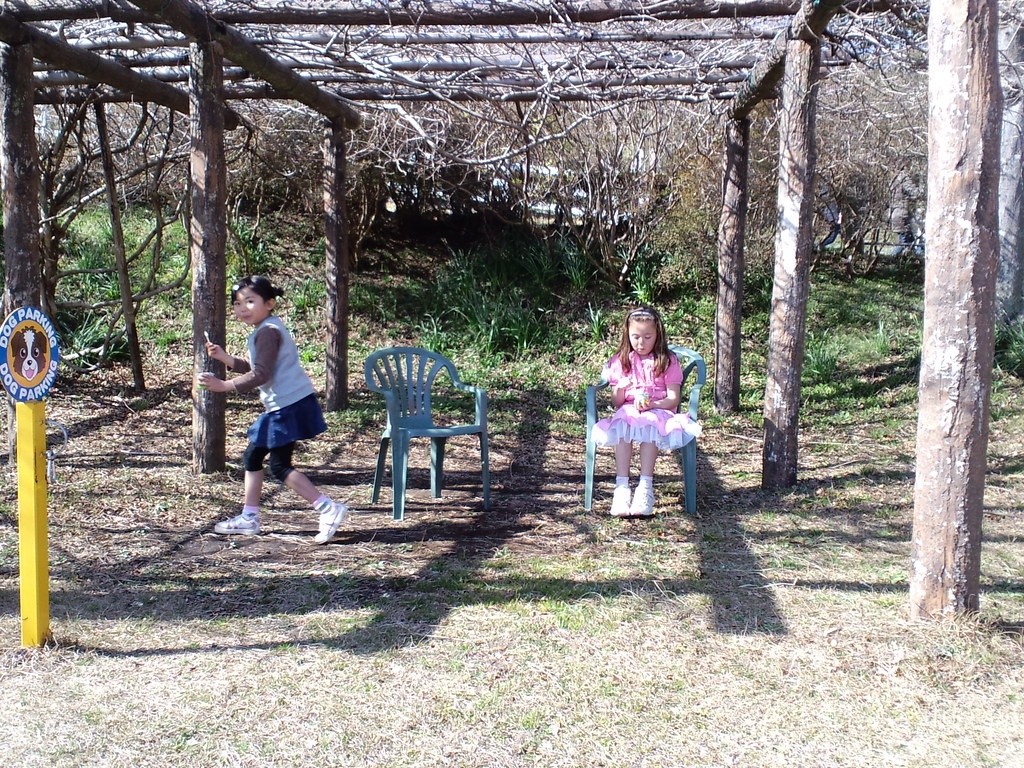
[629,487,655,516]
[314,502,348,545]
[610,488,631,517]
[214,513,260,535]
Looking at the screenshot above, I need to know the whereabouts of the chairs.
[584,345,707,515]
[364,348,492,521]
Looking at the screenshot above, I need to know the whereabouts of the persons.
[889,207,920,258]
[198,274,347,544]
[820,201,844,248]
[592,307,702,517]
